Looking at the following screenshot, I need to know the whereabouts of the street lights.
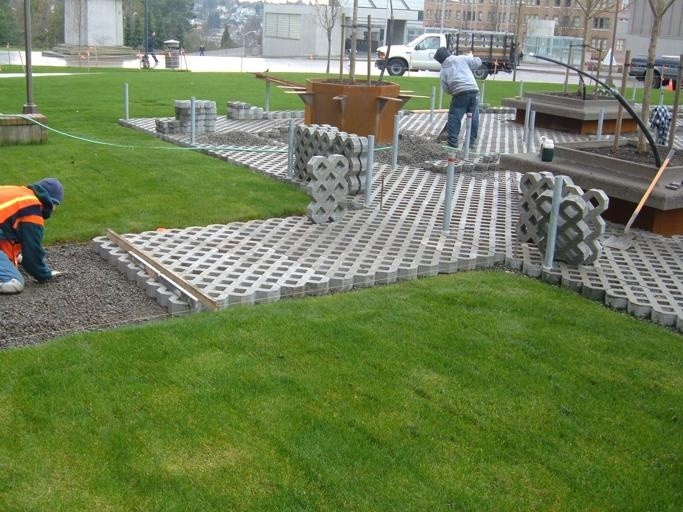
[604,17,629,88]
[240,31,257,73]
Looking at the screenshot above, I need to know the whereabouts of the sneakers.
[440,140,456,148]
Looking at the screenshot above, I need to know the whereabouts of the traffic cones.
[666,78,674,91]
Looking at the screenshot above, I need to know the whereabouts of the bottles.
[540,136,554,162]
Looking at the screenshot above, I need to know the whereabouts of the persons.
[433,47,482,149]
[0,176,77,295]
[142,32,158,64]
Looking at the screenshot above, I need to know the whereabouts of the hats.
[35,177,65,206]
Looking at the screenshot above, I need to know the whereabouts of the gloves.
[51,269,63,277]
[15,254,23,264]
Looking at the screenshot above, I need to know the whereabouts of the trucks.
[628,55,683,90]
[374,30,523,80]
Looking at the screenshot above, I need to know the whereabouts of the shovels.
[603,149,676,250]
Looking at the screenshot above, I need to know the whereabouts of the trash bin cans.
[163,39,180,67]
